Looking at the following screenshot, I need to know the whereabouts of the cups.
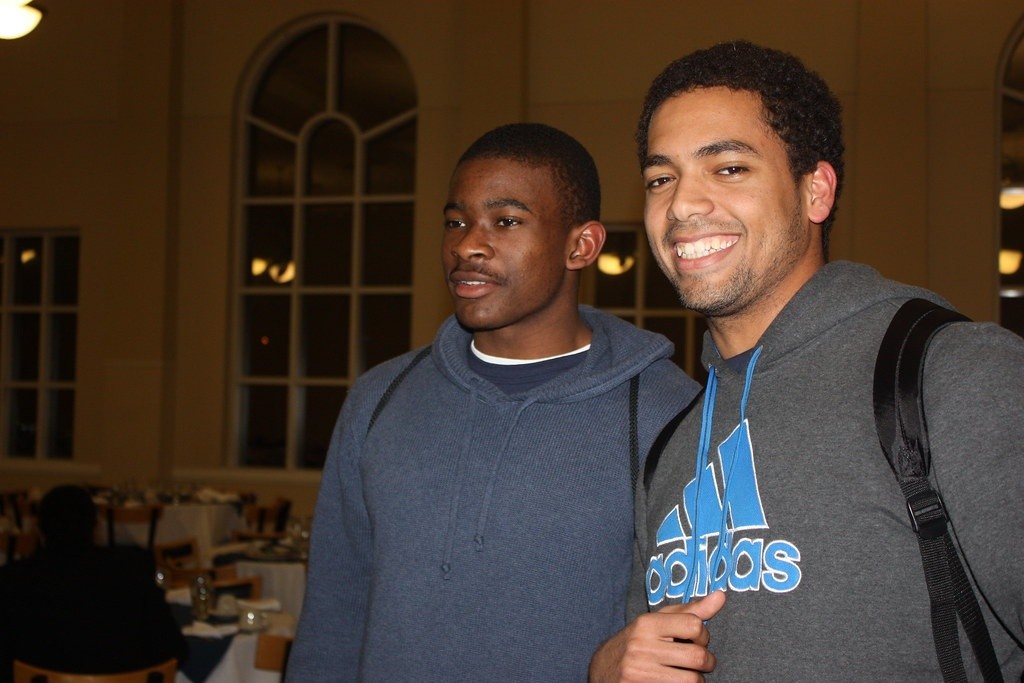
[239,609,269,633]
[181,571,214,623]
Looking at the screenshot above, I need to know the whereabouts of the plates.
[235,599,284,612]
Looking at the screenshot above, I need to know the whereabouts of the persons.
[282,125,704,683]
[589,38,1024,683]
[2,482,189,683]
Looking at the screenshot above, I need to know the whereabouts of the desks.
[0,476,317,683]
